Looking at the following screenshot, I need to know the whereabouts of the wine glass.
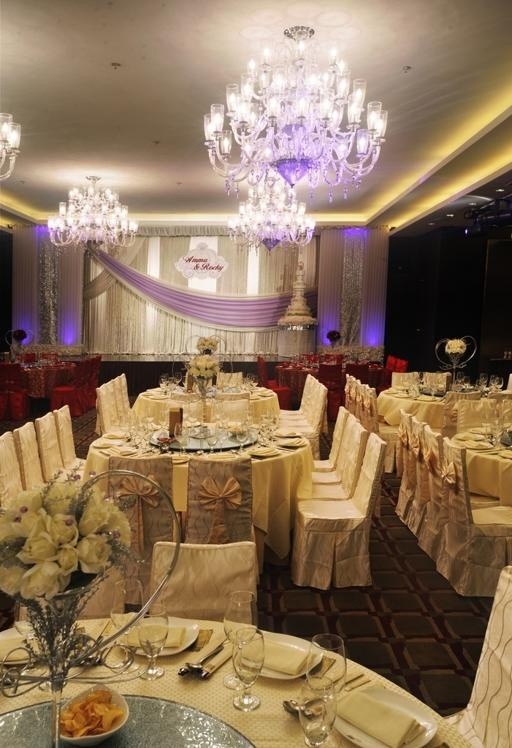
[14,601,47,678]
[306,633,347,703]
[159,372,259,390]
[110,578,143,674]
[232,628,265,712]
[224,591,258,689]
[401,371,504,395]
[298,676,338,747]
[118,400,275,454]
[138,605,168,680]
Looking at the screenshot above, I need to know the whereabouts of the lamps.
[227,169,316,253]
[205,25,389,201]
[47,175,138,248]
[0,110,24,181]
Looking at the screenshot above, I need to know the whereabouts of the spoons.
[283,670,364,713]
[185,646,224,669]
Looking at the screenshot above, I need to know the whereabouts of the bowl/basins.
[60,684,129,746]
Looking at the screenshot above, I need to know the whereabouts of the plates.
[127,617,200,656]
[142,386,274,400]
[334,683,437,748]
[383,389,438,401]
[93,430,307,457]
[455,427,495,450]
[257,632,323,679]
[0,627,37,665]
